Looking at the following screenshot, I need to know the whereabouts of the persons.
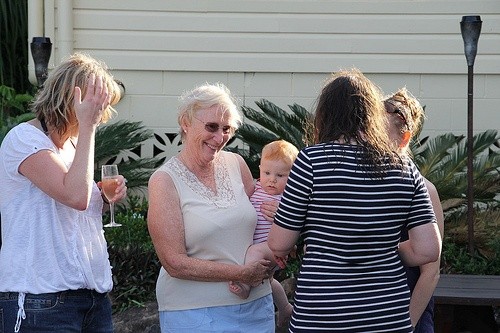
[147,83,280,333]
[268,73,444,333]
[229,140,299,324]
[0,53,127,333]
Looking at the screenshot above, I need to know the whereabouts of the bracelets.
[99,188,109,204]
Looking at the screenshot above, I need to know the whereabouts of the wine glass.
[101,164,122,227]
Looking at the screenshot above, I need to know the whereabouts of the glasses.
[193,116,232,135]
[382,100,412,131]
